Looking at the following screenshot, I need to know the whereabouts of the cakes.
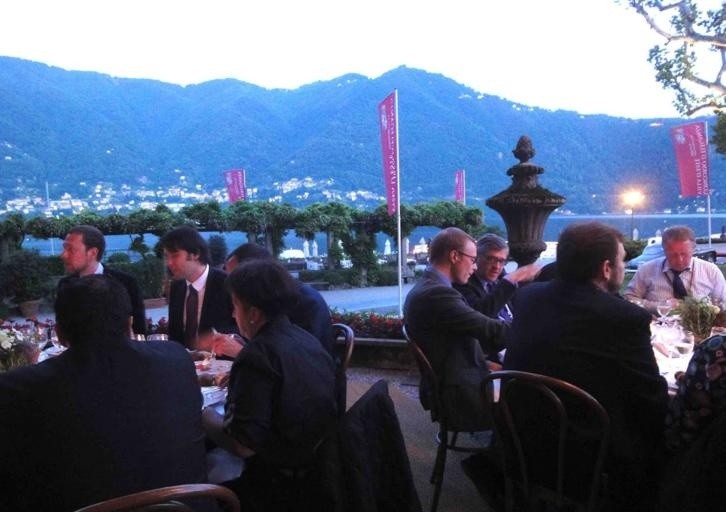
[214,373,227,386]
[199,373,212,386]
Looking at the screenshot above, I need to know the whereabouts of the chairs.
[399,325,506,511]
[481,369,617,511]
[74,482,241,511]
[333,321,355,373]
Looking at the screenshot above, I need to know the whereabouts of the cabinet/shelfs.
[307,380,401,511]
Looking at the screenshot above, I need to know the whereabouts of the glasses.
[483,256,507,266]
[458,251,480,265]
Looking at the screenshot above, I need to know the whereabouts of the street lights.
[624,187,644,242]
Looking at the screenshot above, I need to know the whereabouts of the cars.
[625,241,716,276]
[279,258,427,283]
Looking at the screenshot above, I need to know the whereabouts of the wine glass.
[17,326,169,353]
[620,293,684,361]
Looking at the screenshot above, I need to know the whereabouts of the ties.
[670,270,685,298]
[185,285,199,333]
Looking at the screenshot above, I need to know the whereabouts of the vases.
[692,330,713,345]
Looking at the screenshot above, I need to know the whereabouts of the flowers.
[0,318,50,366]
[673,295,723,335]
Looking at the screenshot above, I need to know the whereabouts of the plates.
[194,360,237,375]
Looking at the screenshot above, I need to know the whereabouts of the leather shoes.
[462,454,507,512]
